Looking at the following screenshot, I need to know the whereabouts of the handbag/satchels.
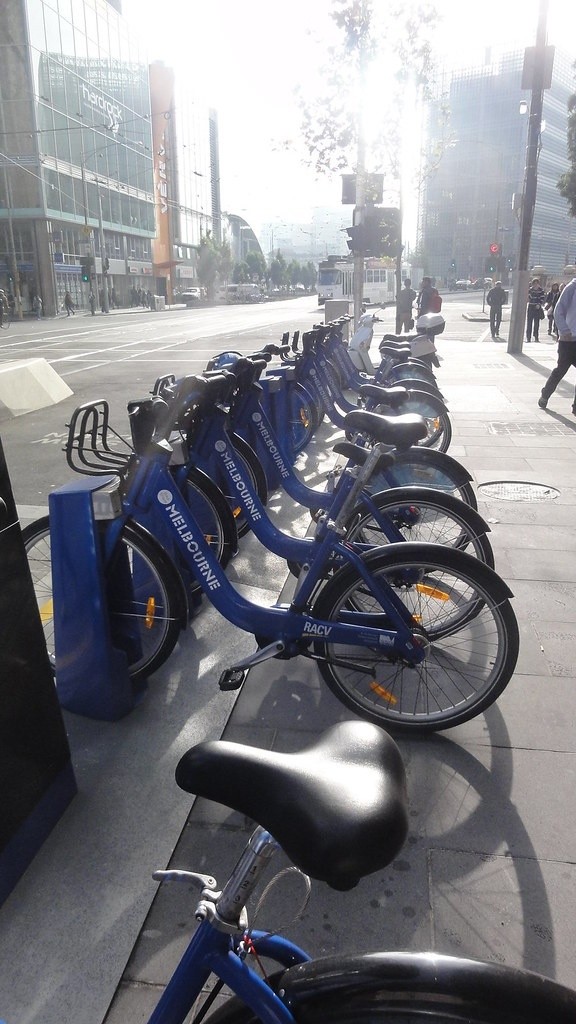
[538,301,553,320]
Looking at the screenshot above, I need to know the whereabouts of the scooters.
[347,301,445,375]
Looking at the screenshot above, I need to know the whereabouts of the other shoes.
[538,396,548,408]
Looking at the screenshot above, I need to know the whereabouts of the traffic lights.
[81,267,89,282]
[346,224,361,251]
[485,255,514,272]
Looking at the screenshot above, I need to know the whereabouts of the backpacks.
[428,288,442,313]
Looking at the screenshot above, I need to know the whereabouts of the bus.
[317,261,408,305]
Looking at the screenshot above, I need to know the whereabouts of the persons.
[65,291,76,317]
[100,287,119,314]
[0,290,42,328]
[130,285,152,310]
[487,281,506,336]
[396,279,415,334]
[537,277,576,413]
[415,277,438,342]
[526,278,566,342]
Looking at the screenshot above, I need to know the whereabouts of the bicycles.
[146,717,576,1024]
[19,369,520,733]
[0,307,10,329]
[168,315,495,639]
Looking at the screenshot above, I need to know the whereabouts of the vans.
[216,284,259,300]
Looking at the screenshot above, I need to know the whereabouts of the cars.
[472,278,501,289]
[456,280,470,290]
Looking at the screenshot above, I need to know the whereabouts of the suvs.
[181,287,201,303]
